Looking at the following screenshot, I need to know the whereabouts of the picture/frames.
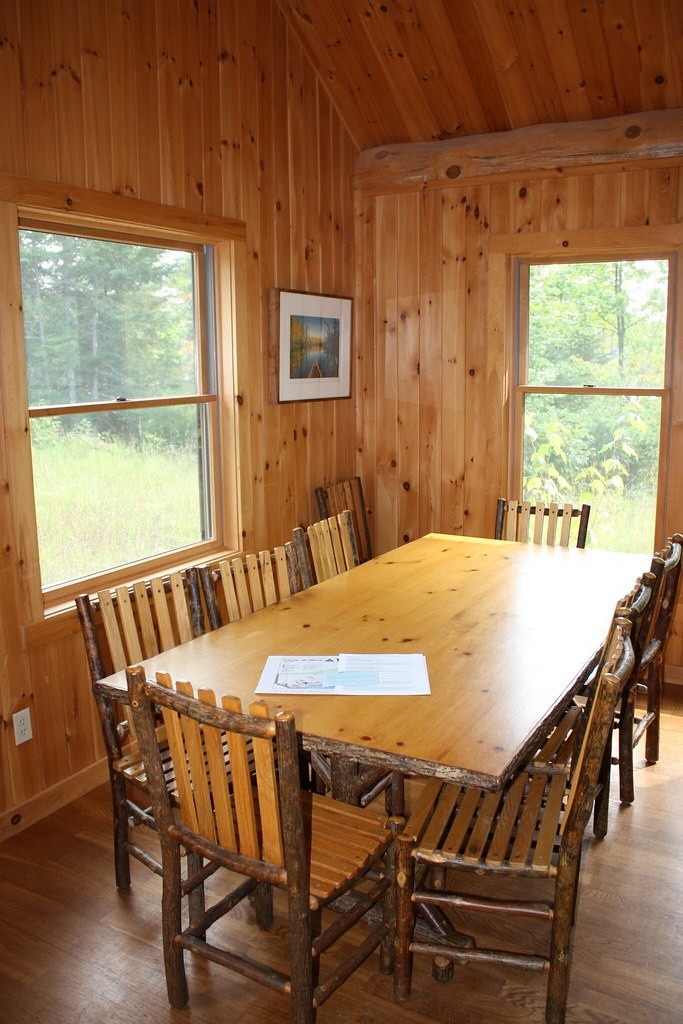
[269,287,353,405]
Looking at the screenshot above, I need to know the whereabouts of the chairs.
[198,477,374,804]
[125,665,405,1024]
[74,568,279,951]
[435,497,683,858]
[391,618,635,1023]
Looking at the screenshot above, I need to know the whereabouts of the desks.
[94,533,656,974]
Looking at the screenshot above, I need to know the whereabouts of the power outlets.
[12,706,33,746]
